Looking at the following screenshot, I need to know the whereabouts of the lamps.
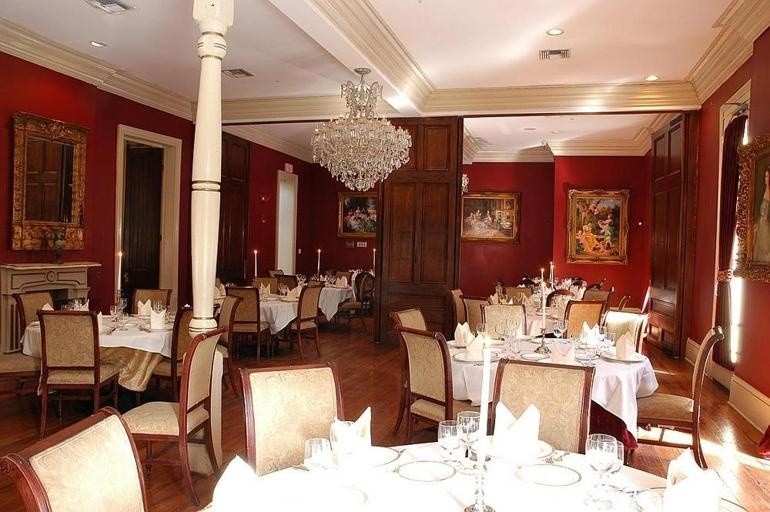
[310,67,412,192]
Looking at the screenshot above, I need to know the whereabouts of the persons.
[344,206,376,232]
[600,213,614,249]
[752,166,770,262]
[583,221,600,248]
[465,200,513,237]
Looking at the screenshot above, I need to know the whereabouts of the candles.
[317,248,322,274]
[254,249,258,277]
[373,248,376,269]
[117,251,123,290]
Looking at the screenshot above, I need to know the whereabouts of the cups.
[304,421,355,477]
[594,326,616,347]
[476,323,489,336]
[61,299,82,311]
[153,300,173,324]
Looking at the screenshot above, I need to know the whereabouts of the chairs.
[0,262,769,512]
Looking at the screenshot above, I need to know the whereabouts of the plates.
[514,463,582,487]
[281,297,299,302]
[371,446,401,467]
[469,435,555,460]
[531,337,553,344]
[396,460,457,483]
[139,321,175,333]
[453,352,498,362]
[520,353,549,361]
[633,486,749,512]
[600,347,646,362]
[446,340,467,348]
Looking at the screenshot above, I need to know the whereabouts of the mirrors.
[11,110,90,251]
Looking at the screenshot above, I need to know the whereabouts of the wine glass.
[277,283,288,297]
[437,410,481,476]
[297,274,306,285]
[553,319,570,344]
[584,434,624,511]
[110,298,129,331]
[326,277,336,285]
[532,276,572,291]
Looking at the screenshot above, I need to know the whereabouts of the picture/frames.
[564,186,629,265]
[459,189,523,245]
[337,191,376,238]
[734,135,770,283]
